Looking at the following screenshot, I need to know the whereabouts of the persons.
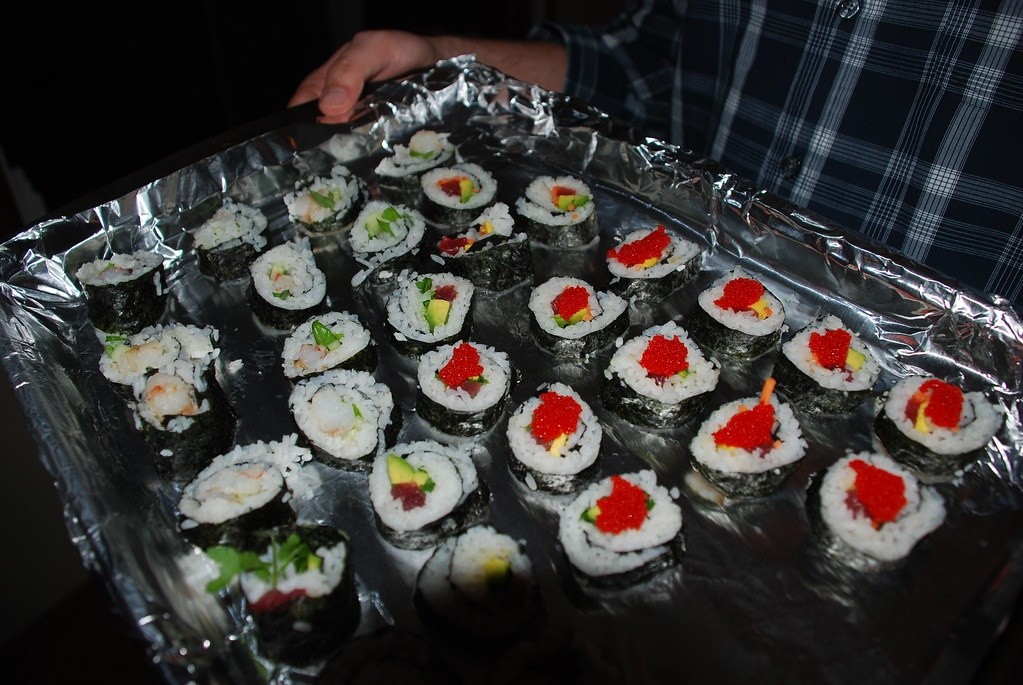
[285,0,1023,317]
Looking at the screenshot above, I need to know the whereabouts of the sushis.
[71,125,1006,668]
